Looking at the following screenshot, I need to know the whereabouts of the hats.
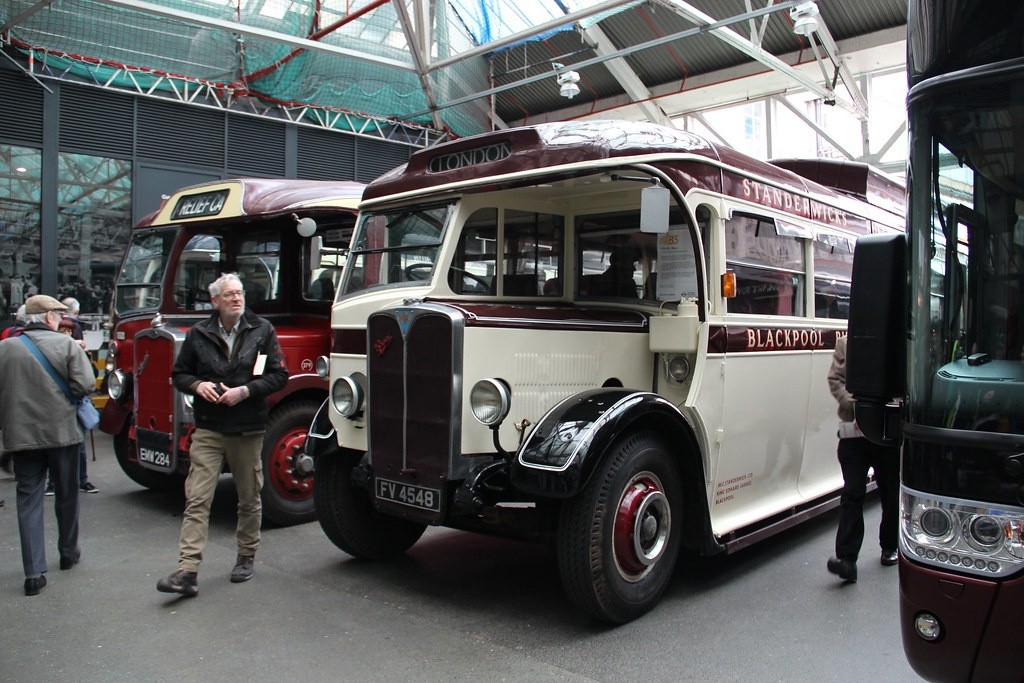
[26,295,68,314]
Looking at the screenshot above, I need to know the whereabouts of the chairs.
[728,273,793,316]
[791,280,840,319]
[543,273,639,298]
[490,273,539,297]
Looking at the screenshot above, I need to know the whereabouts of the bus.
[98,176,365,524]
[307,118,969,625]
[847,0,1024,683]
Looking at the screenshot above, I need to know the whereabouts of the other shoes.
[80,483,99,493]
[24,575,47,595]
[45,483,54,495]
[60,553,80,569]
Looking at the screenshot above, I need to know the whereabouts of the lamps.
[789,1,819,36]
[556,71,581,99]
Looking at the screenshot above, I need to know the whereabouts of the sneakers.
[157,570,197,594]
[230,555,254,581]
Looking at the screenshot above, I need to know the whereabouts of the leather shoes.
[881,549,900,563]
[827,558,857,582]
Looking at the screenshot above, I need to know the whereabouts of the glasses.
[216,290,243,298]
[59,311,65,317]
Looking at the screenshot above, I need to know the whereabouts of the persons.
[602,249,640,298]
[0,295,97,596]
[828,335,905,580]
[0,271,113,315]
[0,298,99,507]
[156,274,289,596]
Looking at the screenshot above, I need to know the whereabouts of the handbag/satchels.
[77,398,100,430]
